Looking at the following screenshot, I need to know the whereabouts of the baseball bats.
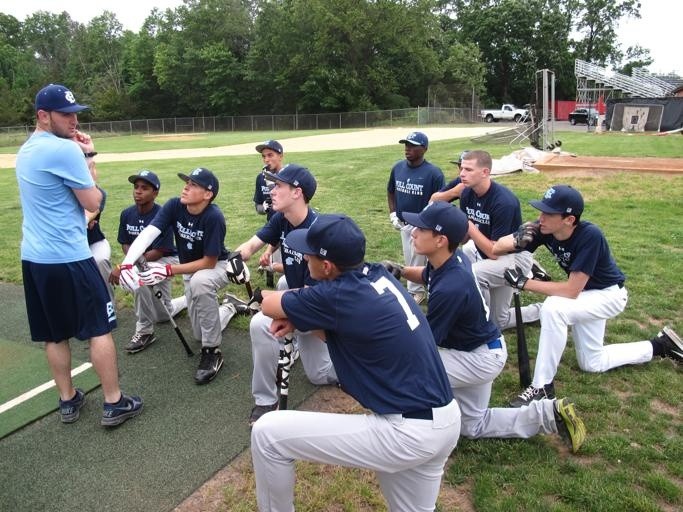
[278,330,292,409]
[138,255,195,357]
[512,286,532,388]
[266,197,274,287]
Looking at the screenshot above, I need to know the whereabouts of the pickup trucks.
[478,103,531,124]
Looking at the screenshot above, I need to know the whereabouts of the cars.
[568,107,606,126]
[521,102,551,121]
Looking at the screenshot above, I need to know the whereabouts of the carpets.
[0,337,101,439]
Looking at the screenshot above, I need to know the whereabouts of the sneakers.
[532,260,551,281]
[195,346,223,384]
[552,398,585,455]
[59,387,86,424]
[100,395,144,426]
[656,326,682,372]
[223,292,248,315]
[248,400,281,427]
[124,334,156,353]
[509,380,556,409]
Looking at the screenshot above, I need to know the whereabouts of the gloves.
[263,197,273,214]
[383,260,404,280]
[389,211,402,229]
[138,262,172,286]
[512,222,539,249]
[225,251,246,285]
[258,262,277,275]
[504,264,529,292]
[119,262,140,291]
[248,287,262,311]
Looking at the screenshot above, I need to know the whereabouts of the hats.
[399,132,428,150]
[256,140,283,155]
[264,164,317,200]
[528,186,583,213]
[178,168,219,197]
[286,214,365,266]
[36,82,90,114]
[402,200,469,242]
[128,170,160,192]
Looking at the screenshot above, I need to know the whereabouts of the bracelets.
[85,151,98,157]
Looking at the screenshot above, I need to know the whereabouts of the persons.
[108,167,261,384]
[251,215,462,512]
[388,133,447,304]
[254,140,284,222]
[381,200,586,454]
[16,84,144,427]
[258,241,290,291]
[492,184,683,408]
[84,184,118,329]
[459,150,541,331]
[118,170,189,354]
[227,163,340,426]
[428,151,466,203]
